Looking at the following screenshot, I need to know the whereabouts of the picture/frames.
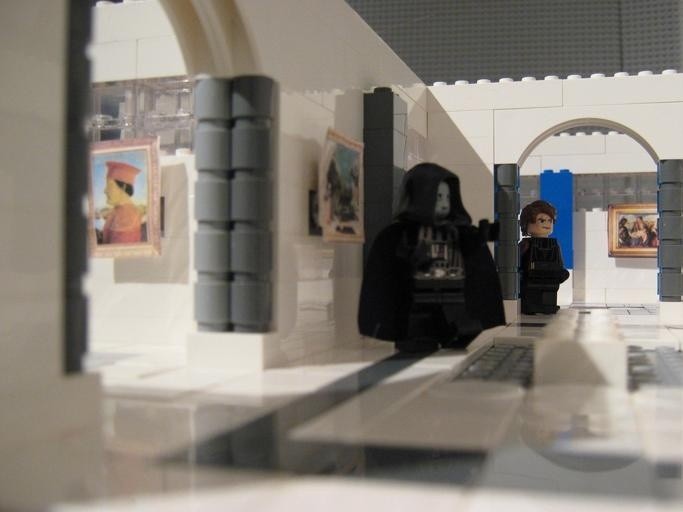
[315,128,365,246]
[608,203,662,259]
[82,136,162,259]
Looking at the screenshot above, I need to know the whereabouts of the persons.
[520,200,570,316]
[359,160,505,355]
[616,217,657,248]
[100,160,143,244]
